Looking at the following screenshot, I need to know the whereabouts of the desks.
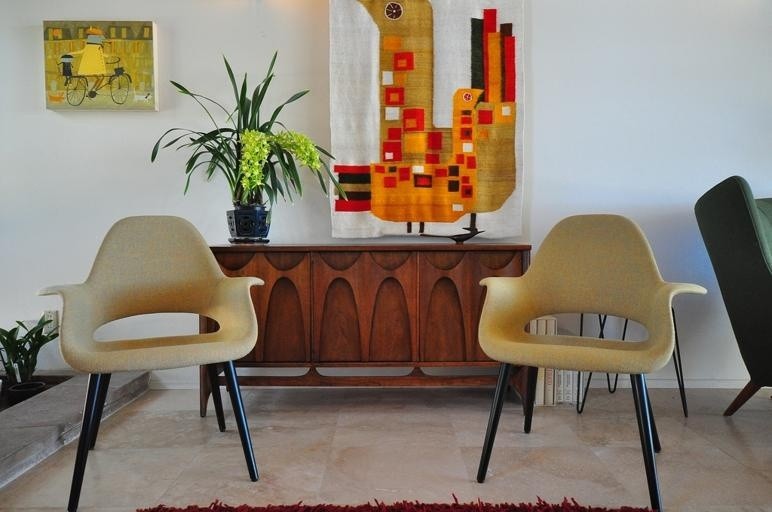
[194,235,534,425]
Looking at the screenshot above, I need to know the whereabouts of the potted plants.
[1,313,62,405]
[147,50,350,239]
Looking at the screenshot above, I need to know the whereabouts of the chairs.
[478,214,707,508]
[694,175,771,415]
[37,210,265,512]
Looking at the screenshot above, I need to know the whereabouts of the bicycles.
[67,56,133,106]
